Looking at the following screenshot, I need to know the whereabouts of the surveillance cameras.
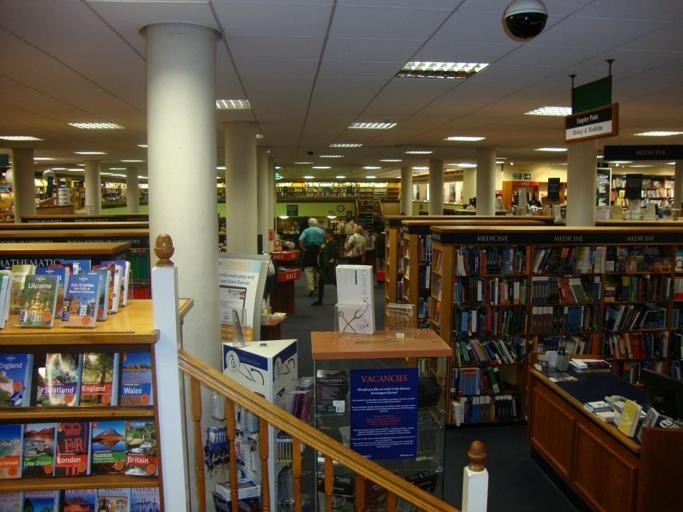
[504,0,548,38]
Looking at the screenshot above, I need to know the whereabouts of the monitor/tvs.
[641,367,683,421]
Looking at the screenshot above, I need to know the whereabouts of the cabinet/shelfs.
[386,215,558,324]
[278,215,351,264]
[401,219,550,378]
[0,214,193,512]
[311,329,452,512]
[428,227,683,429]
[501,171,682,226]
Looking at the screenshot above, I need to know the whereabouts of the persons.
[333,216,384,268]
[298,218,328,297]
[310,227,341,305]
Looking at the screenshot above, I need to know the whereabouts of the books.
[616,399,643,440]
[334,263,375,335]
[0,488,160,511]
[531,247,608,366]
[0,352,154,409]
[0,261,130,329]
[0,420,158,481]
[595,177,682,221]
[508,203,566,222]
[604,245,673,384]
[452,245,526,427]
[669,250,682,379]
[383,227,442,337]
[635,405,661,445]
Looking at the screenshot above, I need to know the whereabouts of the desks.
[532,367,683,512]
